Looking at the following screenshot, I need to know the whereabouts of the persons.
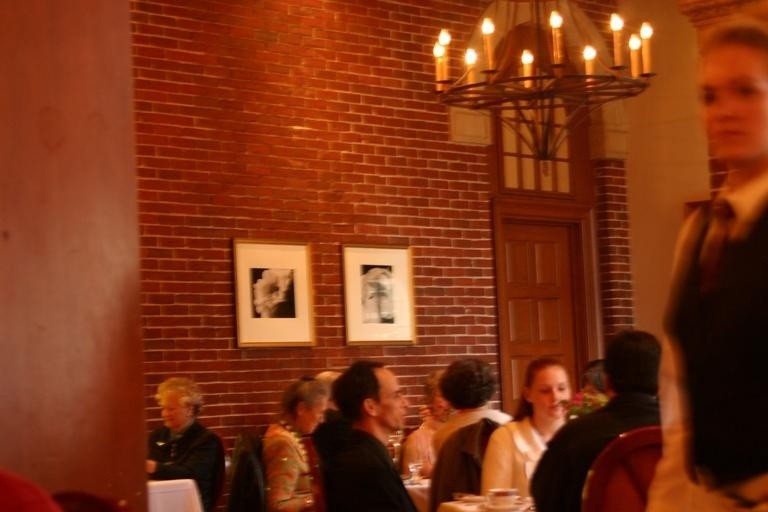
[531,331,662,511]
[262,358,571,512]
[0,466,61,512]
[144,376,227,511]
[657,17,767,512]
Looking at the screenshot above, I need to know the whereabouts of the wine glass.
[408,448,423,483]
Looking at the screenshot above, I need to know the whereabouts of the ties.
[697,200,733,298]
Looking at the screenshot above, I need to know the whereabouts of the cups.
[484,486,522,506]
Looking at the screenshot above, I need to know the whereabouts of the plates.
[478,502,531,511]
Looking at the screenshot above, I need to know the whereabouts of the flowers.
[558,383,614,417]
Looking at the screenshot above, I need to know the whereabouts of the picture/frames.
[233,236,316,349]
[341,242,418,348]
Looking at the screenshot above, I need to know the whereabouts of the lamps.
[432,0,657,176]
